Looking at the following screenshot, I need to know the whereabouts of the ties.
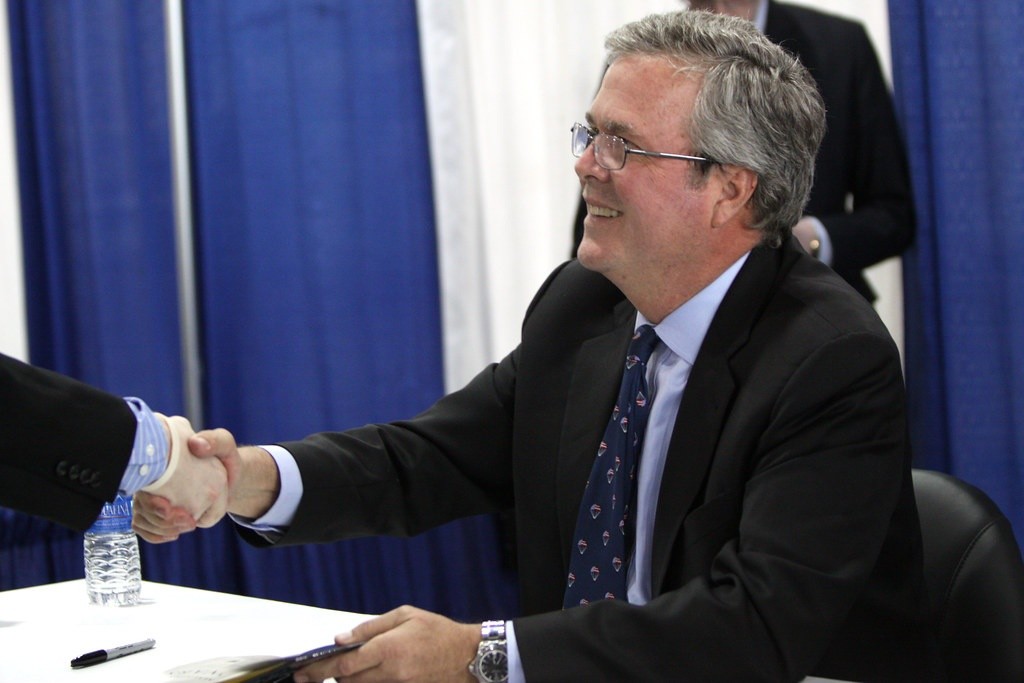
[561,322,661,609]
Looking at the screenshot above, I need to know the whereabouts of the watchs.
[469,618,511,683]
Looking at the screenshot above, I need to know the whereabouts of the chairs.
[910,467,1024,683]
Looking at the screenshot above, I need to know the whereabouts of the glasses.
[569,120,712,171]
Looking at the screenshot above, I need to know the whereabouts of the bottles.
[84,490,142,607]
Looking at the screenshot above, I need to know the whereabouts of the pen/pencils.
[71,639,155,669]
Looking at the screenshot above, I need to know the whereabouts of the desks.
[0,577,395,683]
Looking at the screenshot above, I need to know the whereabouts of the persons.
[0,353,230,533]
[569,2,915,314]
[134,11,926,683]
